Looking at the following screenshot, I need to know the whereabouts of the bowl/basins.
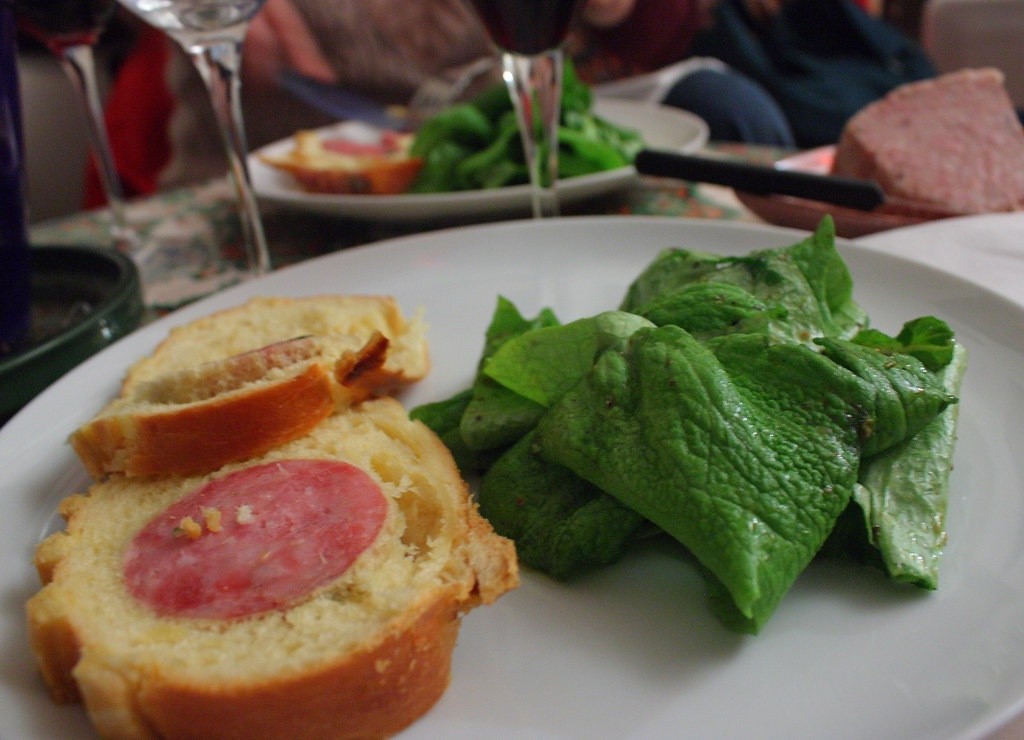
[0,241,140,428]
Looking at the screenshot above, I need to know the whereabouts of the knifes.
[634,148,960,218]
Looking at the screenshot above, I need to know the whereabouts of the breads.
[256,121,428,192]
[24,292,521,740]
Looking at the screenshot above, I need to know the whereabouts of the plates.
[247,96,710,227]
[0,213,1024,740]
[735,146,928,239]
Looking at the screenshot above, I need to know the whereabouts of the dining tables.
[0,143,1024,740]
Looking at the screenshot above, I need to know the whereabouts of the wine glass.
[465,0,583,219]
[120,0,272,281]
[5,0,136,250]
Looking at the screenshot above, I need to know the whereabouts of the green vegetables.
[407,66,644,175]
[409,215,967,637]
